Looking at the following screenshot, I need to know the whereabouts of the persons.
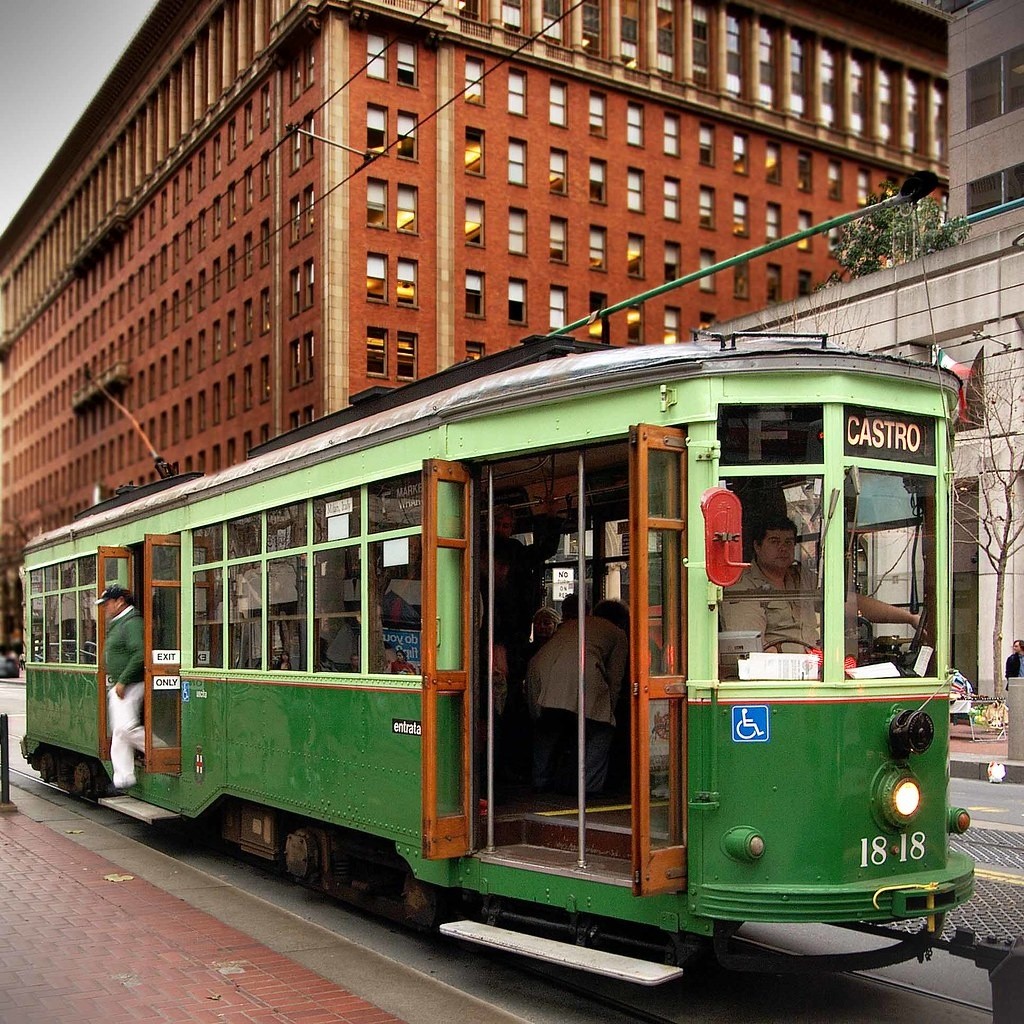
[382,537,422,623]
[481,502,659,794]
[721,515,927,664]
[1005,640,1024,689]
[18,653,26,671]
[246,641,417,677]
[93,583,172,794]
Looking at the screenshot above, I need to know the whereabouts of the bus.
[17,327,979,1003]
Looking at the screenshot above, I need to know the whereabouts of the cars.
[0,656,19,679]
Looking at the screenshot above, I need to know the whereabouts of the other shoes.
[107,783,118,790]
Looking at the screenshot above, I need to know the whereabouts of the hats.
[94,586,131,604]
